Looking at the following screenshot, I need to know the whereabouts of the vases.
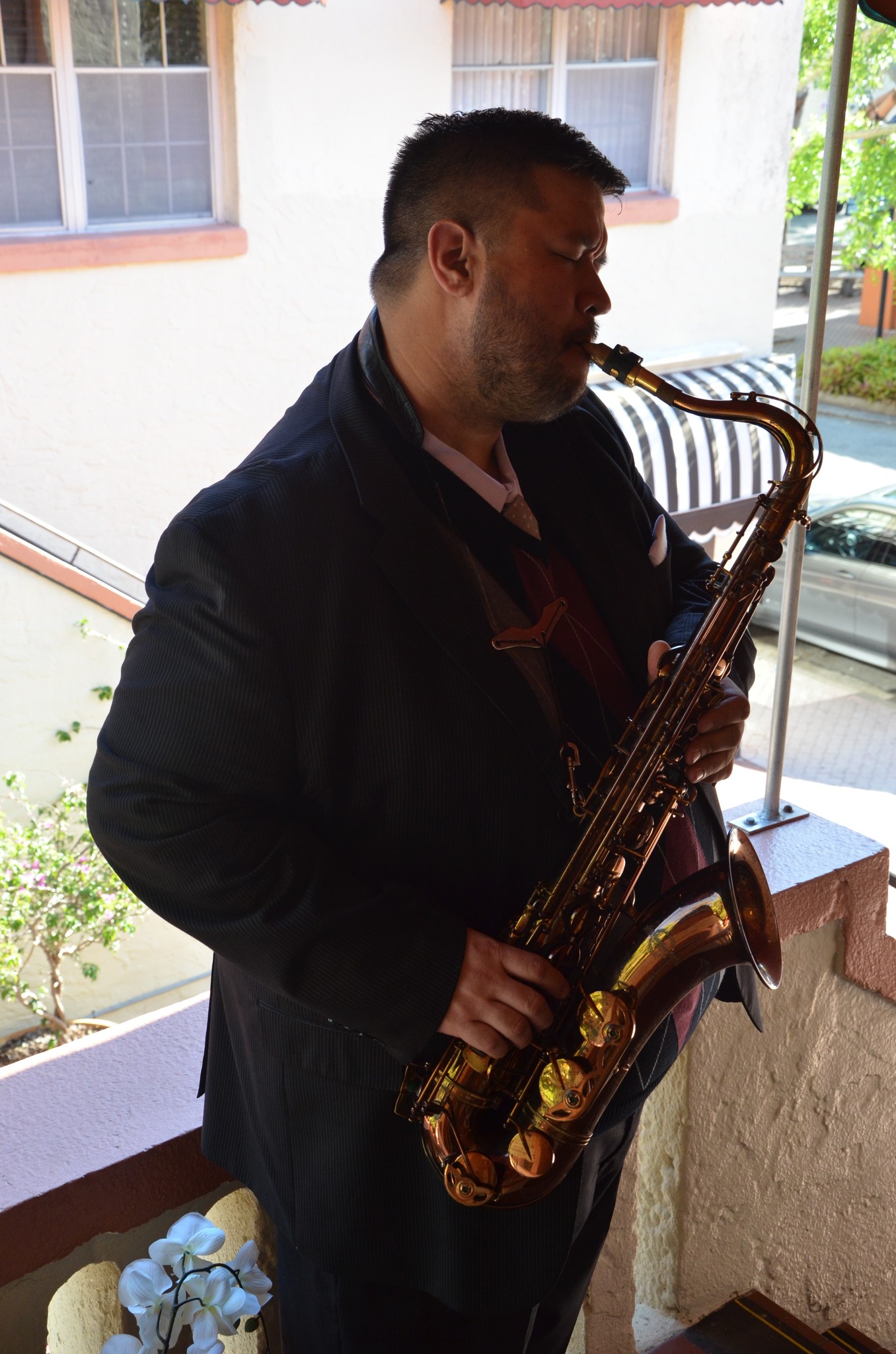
[0,1018,118,1068]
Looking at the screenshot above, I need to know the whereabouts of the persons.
[82,108,757,1354]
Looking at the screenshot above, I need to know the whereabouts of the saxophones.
[394,342,825,1208]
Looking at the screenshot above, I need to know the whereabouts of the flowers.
[1,620,148,1047]
[101,1212,273,1354]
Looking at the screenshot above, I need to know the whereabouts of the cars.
[740,488,896,682]
